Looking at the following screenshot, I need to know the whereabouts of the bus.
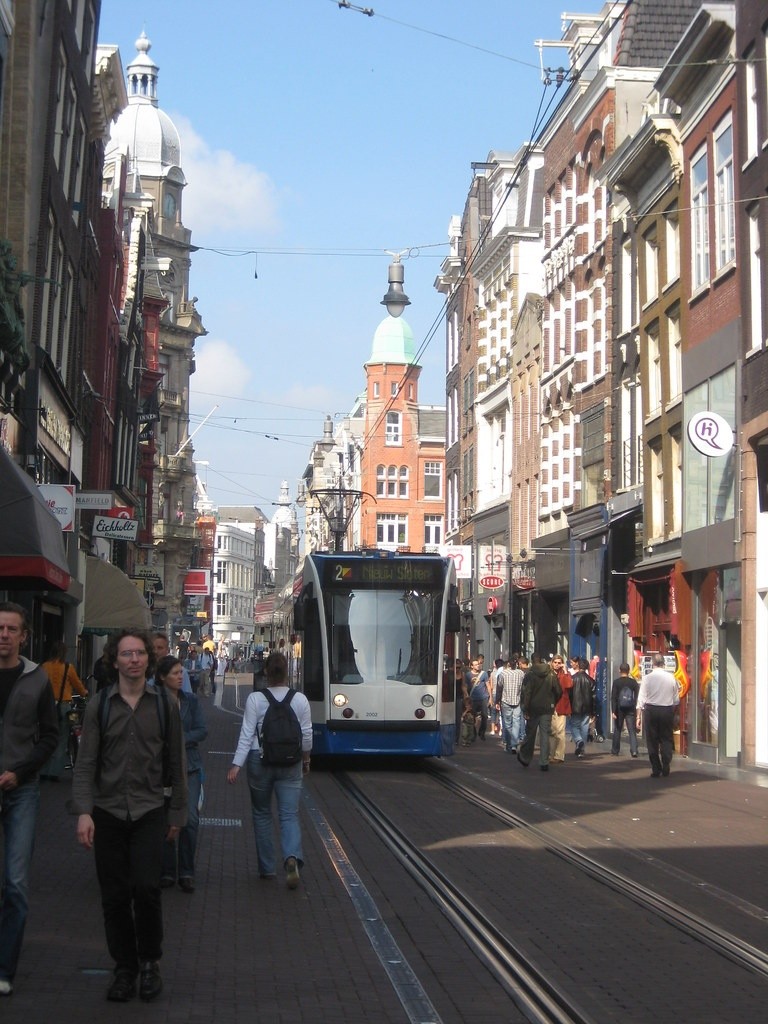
[268,488,461,755]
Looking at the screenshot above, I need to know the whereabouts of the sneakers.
[107,971,136,1001]
[139,961,163,999]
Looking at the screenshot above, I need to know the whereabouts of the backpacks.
[619,678,633,709]
[254,688,303,766]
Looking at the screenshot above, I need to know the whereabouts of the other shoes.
[178,878,195,892]
[286,858,300,889]
[0,978,14,995]
[460,729,670,777]
[39,774,58,782]
[259,873,275,879]
[159,874,174,888]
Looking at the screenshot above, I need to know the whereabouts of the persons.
[227,653,313,888]
[546,656,574,765]
[456,653,529,747]
[636,655,680,777]
[567,657,589,676]
[495,658,525,752]
[40,640,88,770]
[611,663,639,756]
[70,632,189,1000]
[155,656,210,892]
[570,659,598,759]
[517,653,563,771]
[0,602,59,993]
[94,635,217,698]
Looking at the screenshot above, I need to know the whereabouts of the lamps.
[381,250,412,316]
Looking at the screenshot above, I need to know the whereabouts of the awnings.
[1,446,71,594]
[83,555,156,631]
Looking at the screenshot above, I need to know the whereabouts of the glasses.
[553,661,561,664]
[120,650,146,657]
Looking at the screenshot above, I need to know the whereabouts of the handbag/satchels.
[56,703,63,721]
